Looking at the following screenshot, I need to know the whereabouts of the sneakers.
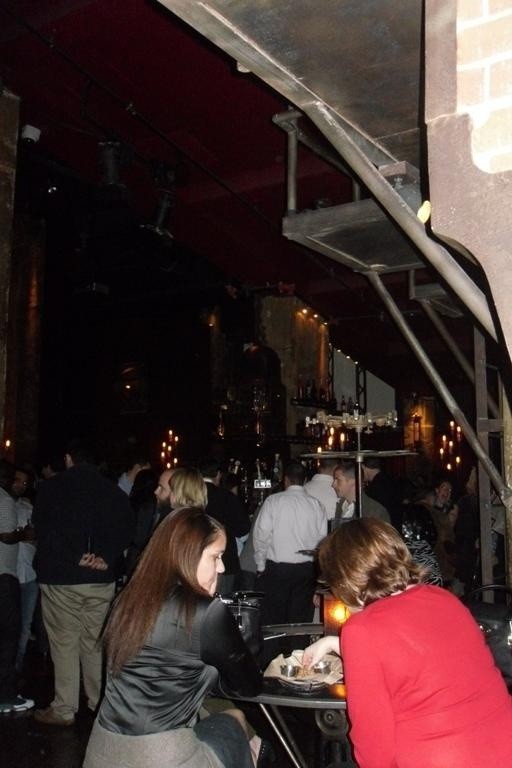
[0,693,36,714]
[34,707,75,726]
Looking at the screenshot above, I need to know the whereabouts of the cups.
[280,664,301,677]
[314,660,331,674]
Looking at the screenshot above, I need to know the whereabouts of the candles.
[325,426,346,452]
[160,429,180,469]
[434,420,464,484]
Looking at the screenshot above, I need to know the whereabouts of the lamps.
[317,586,351,637]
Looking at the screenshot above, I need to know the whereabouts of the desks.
[208,635,359,768]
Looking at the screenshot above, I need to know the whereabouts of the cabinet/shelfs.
[224,409,274,445]
[285,395,336,445]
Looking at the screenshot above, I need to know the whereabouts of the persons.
[1,441,512,767]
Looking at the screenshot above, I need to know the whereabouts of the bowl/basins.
[274,655,342,695]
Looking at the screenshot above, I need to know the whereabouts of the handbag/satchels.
[215,589,265,660]
[463,583,512,682]
